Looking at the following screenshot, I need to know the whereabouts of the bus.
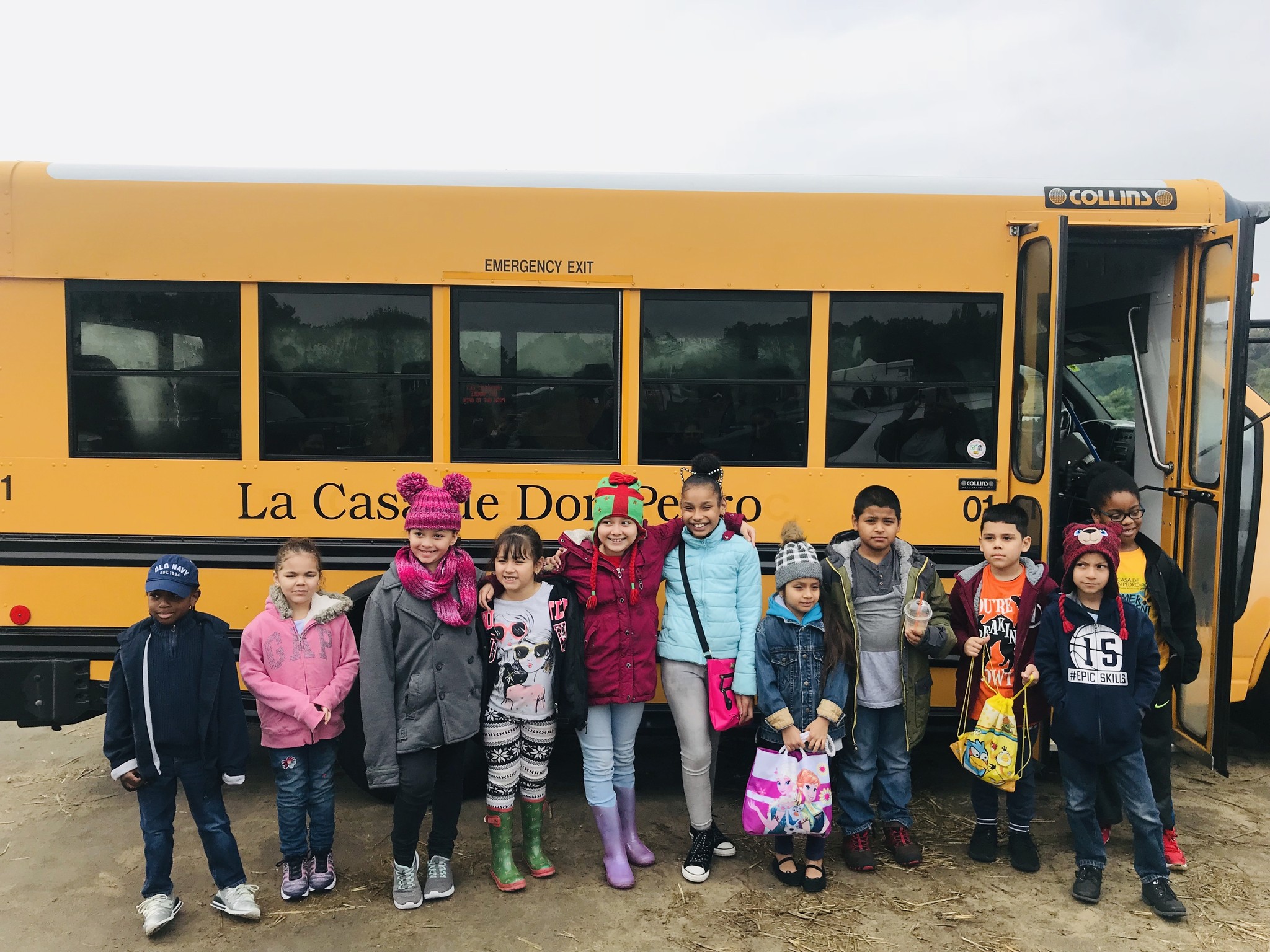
[0,158,1270,803]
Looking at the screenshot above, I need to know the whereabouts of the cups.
[903,598,933,636]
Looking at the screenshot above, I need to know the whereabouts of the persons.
[755,519,856,892]
[478,472,754,890]
[102,554,261,937]
[820,485,957,872]
[1079,467,1202,873]
[474,525,590,894]
[948,504,1059,872]
[1033,520,1186,919]
[239,536,360,901]
[360,472,487,909]
[544,454,760,882]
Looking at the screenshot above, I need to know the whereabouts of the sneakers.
[209,884,260,919]
[1163,826,1188,869]
[842,821,922,871]
[967,825,1041,873]
[393,850,455,909]
[275,849,310,902]
[1099,825,1112,845]
[136,894,184,935]
[307,850,336,893]
[681,820,737,885]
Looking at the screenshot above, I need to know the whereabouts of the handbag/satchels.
[741,730,835,838]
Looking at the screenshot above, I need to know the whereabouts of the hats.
[396,473,471,529]
[145,555,200,598]
[774,521,823,592]
[586,471,647,610]
[1059,522,1128,640]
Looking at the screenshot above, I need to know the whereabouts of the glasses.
[1097,503,1145,521]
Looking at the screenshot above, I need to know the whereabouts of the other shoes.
[1142,878,1187,917]
[1072,865,1103,902]
[771,855,827,893]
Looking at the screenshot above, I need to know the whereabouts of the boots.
[484,807,527,891]
[519,795,556,876]
[613,786,655,867]
[591,800,634,889]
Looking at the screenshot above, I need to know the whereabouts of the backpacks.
[950,642,1035,792]
